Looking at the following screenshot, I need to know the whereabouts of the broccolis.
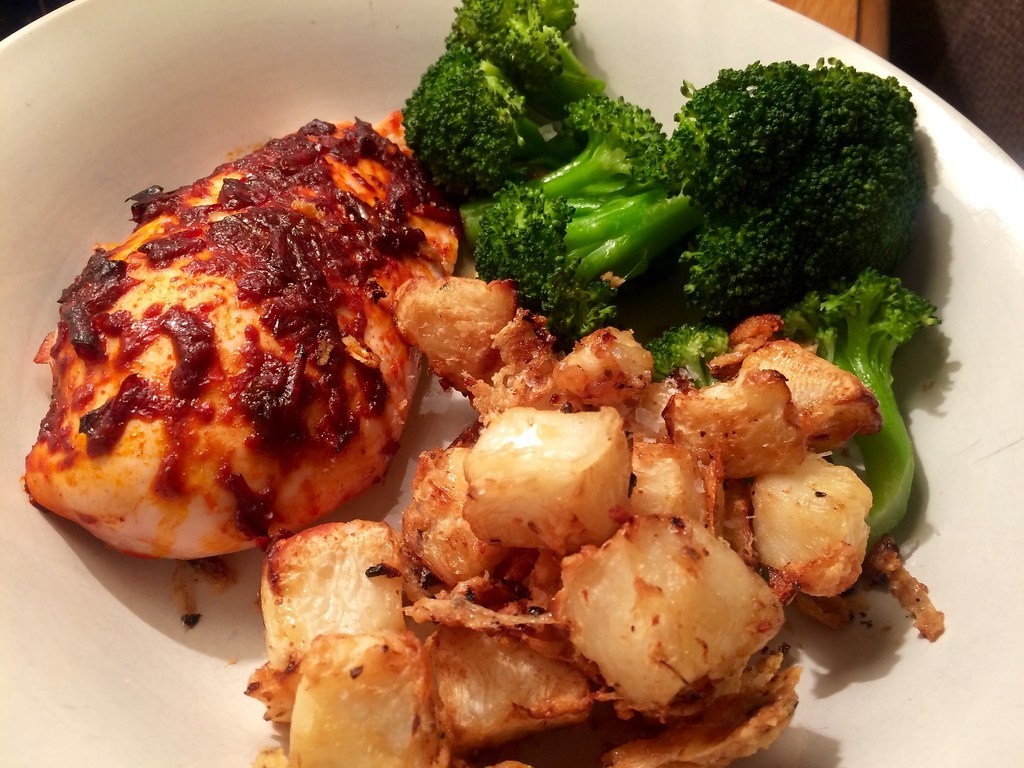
[398,0,943,548]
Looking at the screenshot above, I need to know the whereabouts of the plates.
[0,0,1021,768]
